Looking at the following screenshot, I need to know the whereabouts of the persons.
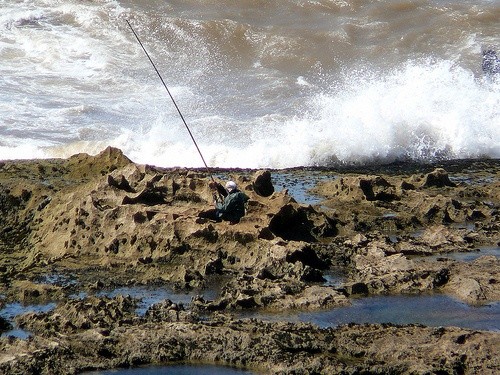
[210,181,248,223]
